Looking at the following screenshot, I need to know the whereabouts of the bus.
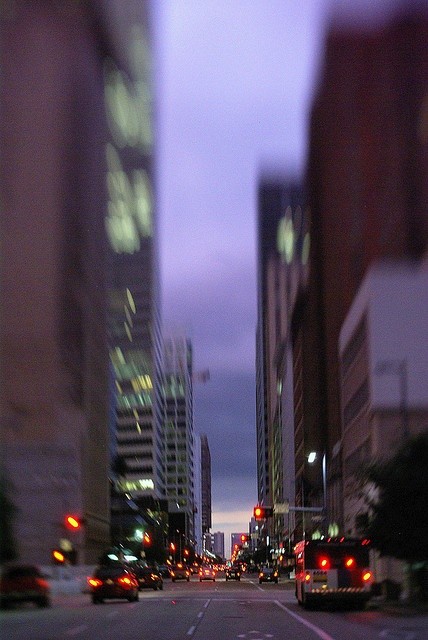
[294,538,372,610]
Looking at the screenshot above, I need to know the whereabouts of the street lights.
[307,451,329,534]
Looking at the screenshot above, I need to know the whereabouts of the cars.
[92,565,140,605]
[198,567,216,583]
[225,569,240,582]
[1,565,52,610]
[258,567,279,584]
[170,564,190,583]
[134,565,163,592]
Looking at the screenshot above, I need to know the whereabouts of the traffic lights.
[253,506,273,519]
